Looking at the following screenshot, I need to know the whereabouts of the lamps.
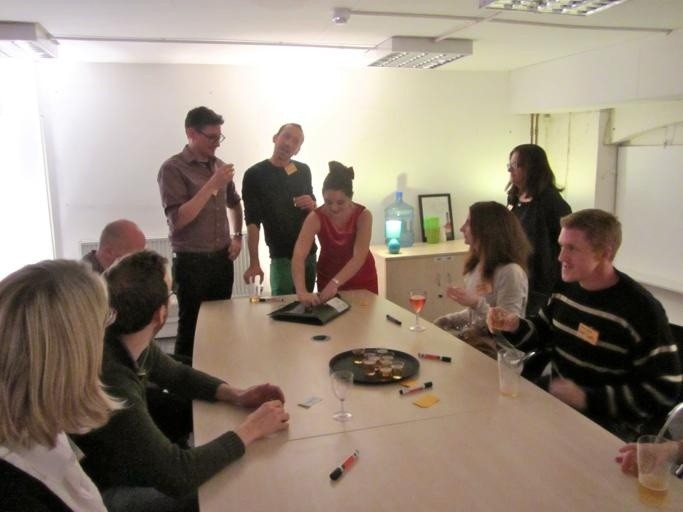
[2,20,59,61]
[363,36,475,73]
[474,0,624,18]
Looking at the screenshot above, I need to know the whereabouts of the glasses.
[165,282,181,298]
[506,161,522,168]
[195,127,225,144]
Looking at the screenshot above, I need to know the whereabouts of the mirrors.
[416,192,457,242]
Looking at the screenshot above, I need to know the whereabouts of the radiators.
[77,232,264,323]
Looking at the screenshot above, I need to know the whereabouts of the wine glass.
[406,287,429,330]
[331,369,352,424]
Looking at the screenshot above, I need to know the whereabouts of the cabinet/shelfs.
[369,237,470,321]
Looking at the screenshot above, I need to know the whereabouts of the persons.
[155,105,244,357]
[431,200,534,360]
[78,219,147,277]
[290,161,380,308]
[64,250,291,512]
[613,432,683,480]
[0,258,129,512]
[484,207,682,443]
[502,143,572,318]
[242,124,318,294]
[240,464,241,466]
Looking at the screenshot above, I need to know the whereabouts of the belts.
[170,251,199,258]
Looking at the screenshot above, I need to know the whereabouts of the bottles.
[443,212,453,240]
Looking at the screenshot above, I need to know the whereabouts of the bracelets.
[230,232,242,238]
[330,278,342,289]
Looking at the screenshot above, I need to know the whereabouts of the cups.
[637,434,669,506]
[293,196,297,207]
[426,217,439,244]
[222,163,233,174]
[493,311,503,334]
[498,347,524,401]
[248,282,263,302]
[350,345,403,379]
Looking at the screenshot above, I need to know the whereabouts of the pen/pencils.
[399,382,432,394]
[417,353,452,361]
[329,447,360,480]
[387,315,401,325]
[259,298,283,301]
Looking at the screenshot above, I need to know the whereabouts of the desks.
[186,286,682,511]
